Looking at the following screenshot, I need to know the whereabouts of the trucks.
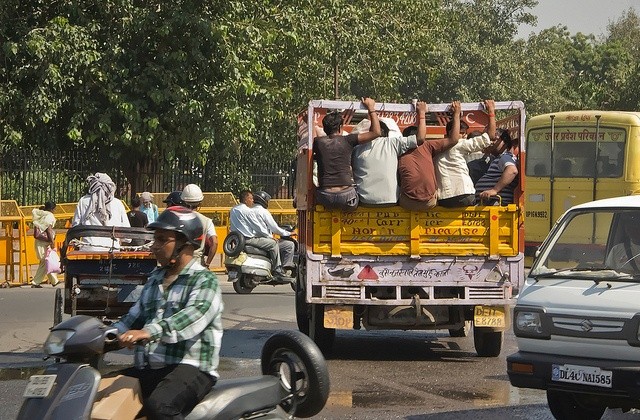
[296,100,525,360]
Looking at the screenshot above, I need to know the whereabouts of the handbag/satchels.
[34,226,55,241]
[44,248,61,275]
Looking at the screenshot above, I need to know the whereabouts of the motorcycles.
[17,315,330,420]
[222,225,299,294]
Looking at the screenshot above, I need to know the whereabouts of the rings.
[127,335,133,339]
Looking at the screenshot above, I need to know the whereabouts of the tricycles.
[49,223,155,364]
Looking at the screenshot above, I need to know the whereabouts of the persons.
[463,130,496,187]
[162,191,190,209]
[605,213,640,274]
[30,200,61,288]
[250,191,297,270]
[603,163,620,177]
[555,159,573,177]
[312,97,382,211]
[230,189,288,278]
[109,205,224,420]
[348,100,427,205]
[432,99,495,209]
[181,184,217,271]
[474,129,520,206]
[617,142,626,176]
[581,148,602,177]
[397,100,461,211]
[72,172,132,252]
[534,163,546,176]
[122,199,150,246]
[139,192,159,226]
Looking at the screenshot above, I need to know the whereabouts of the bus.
[525,109,640,272]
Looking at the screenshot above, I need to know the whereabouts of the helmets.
[181,184,204,202]
[146,206,204,249]
[253,191,271,209]
[162,191,183,204]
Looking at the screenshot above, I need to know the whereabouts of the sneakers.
[273,266,287,277]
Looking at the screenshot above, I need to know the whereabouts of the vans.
[506,195,639,420]
